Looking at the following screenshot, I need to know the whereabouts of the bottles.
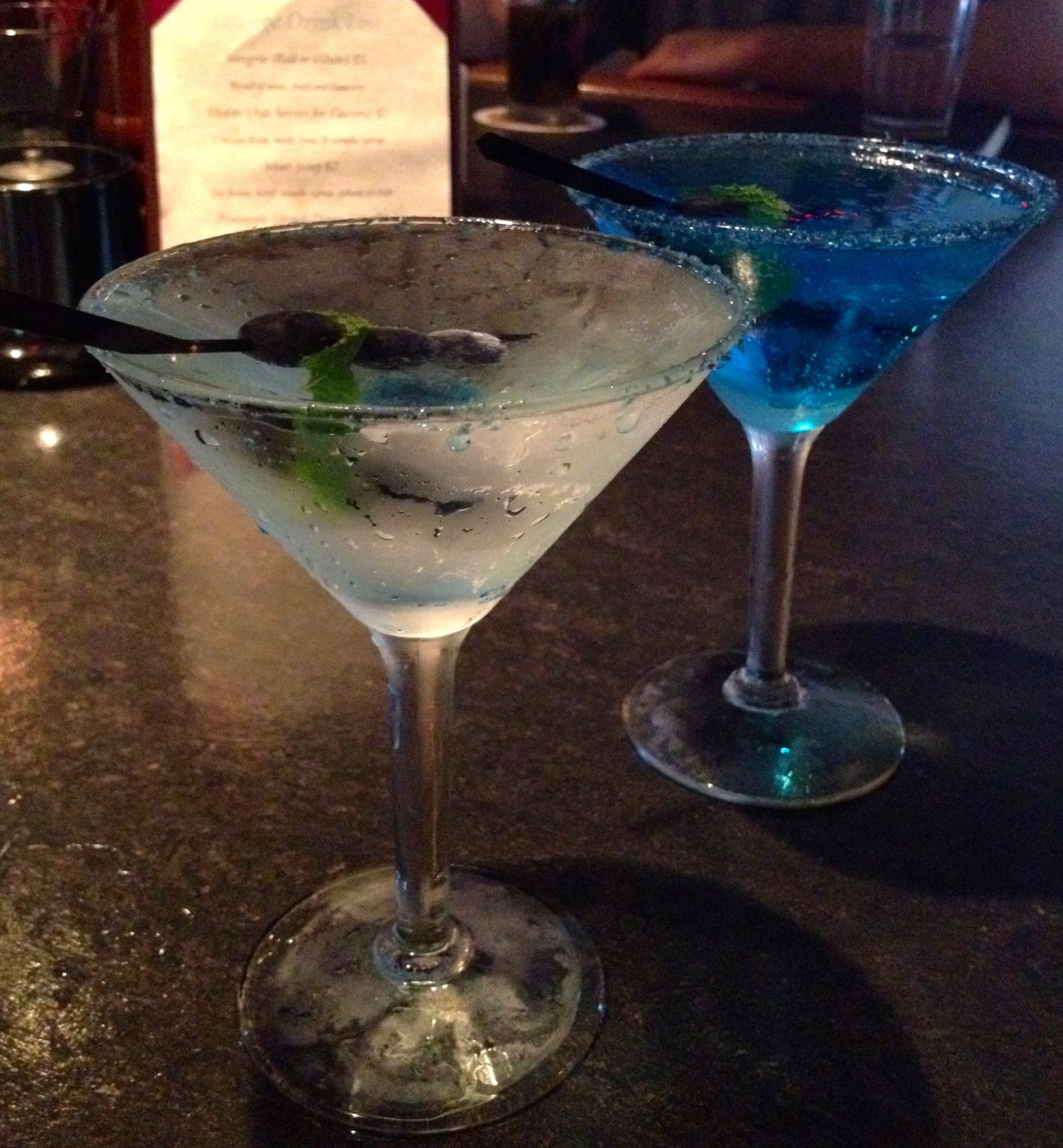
[1,147,72,184]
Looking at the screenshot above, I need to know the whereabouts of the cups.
[862,0,980,140]
[508,0,588,120]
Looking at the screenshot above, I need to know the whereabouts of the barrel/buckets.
[1,141,137,346]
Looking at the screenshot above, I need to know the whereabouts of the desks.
[0,229,1063,1148]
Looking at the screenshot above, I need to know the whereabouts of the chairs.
[464,62,1063,226]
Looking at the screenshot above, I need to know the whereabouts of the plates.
[473,104,603,134]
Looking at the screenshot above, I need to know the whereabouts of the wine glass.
[81,213,760,1136]
[566,129,1059,807]
